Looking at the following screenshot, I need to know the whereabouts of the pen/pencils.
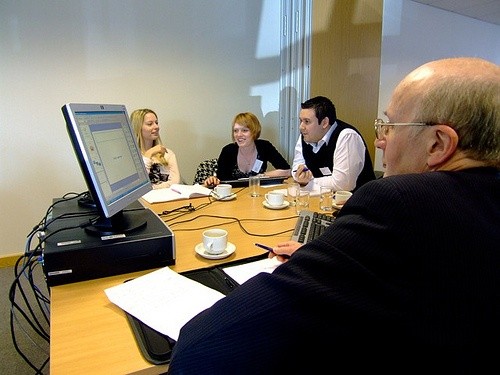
[293,168,308,172]
[255,243,291,259]
[171,189,181,194]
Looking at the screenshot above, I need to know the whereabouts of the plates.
[333,197,344,210]
[195,241,236,260]
[212,192,237,201]
[263,200,288,209]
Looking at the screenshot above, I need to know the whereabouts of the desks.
[50,177,336,375]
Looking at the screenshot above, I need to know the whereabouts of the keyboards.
[288,210,336,245]
[219,177,284,188]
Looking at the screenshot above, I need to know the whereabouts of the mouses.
[208,182,216,189]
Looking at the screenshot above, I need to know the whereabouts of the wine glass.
[287,182,300,206]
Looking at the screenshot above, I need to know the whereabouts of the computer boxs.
[43,197,177,288]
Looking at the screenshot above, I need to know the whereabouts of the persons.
[165,56,500,375]
[130,109,180,190]
[291,96,376,194]
[205,112,292,187]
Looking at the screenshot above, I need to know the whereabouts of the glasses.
[374,119,461,147]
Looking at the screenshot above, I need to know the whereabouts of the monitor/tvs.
[61,103,153,237]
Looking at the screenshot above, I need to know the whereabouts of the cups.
[296,190,310,215]
[249,176,260,198]
[336,191,349,205]
[202,229,228,254]
[214,184,232,198]
[265,191,285,205]
[320,186,334,211]
